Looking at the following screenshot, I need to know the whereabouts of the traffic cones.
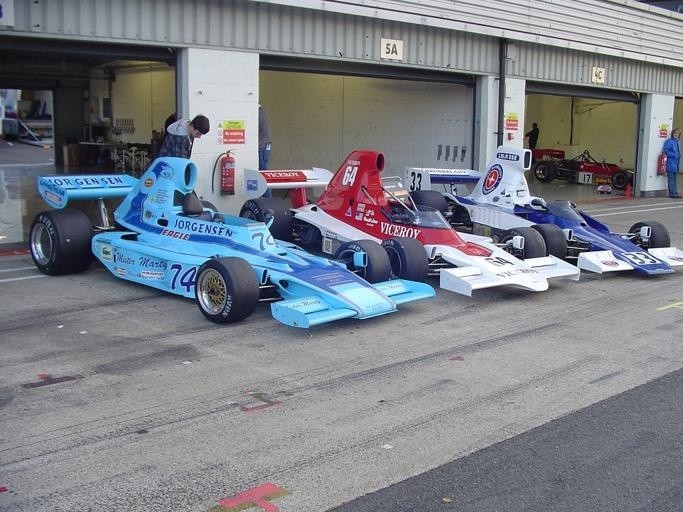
[624,184,633,197]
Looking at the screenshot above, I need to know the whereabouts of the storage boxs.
[596,184,611,195]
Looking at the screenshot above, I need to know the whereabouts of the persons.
[524,123,539,163]
[158,114,211,209]
[661,128,682,198]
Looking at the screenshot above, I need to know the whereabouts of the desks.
[79,141,120,167]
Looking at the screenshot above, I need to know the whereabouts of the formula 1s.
[531,147,632,191]
[232,146,585,306]
[397,141,683,282]
[20,152,438,335]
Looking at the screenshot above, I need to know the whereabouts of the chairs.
[409,187,468,228]
[175,187,210,219]
